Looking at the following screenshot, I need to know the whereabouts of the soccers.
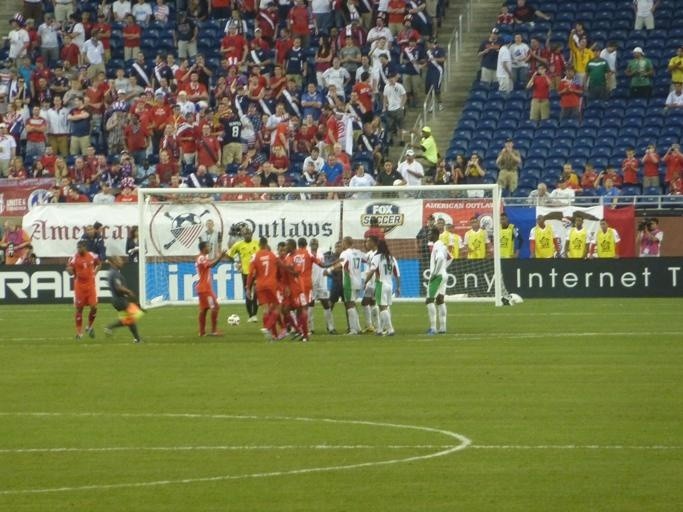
[228,314,240,327]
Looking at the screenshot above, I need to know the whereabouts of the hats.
[117,88,127,95]
[632,46,645,55]
[9,12,24,27]
[422,125,431,134]
[405,148,414,157]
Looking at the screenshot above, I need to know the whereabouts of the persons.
[1,0,682,210]
[2,214,664,343]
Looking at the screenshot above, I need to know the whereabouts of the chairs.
[0,0,682,207]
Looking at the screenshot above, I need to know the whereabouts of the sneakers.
[105,326,112,336]
[131,336,144,345]
[260,323,450,344]
[85,325,95,339]
